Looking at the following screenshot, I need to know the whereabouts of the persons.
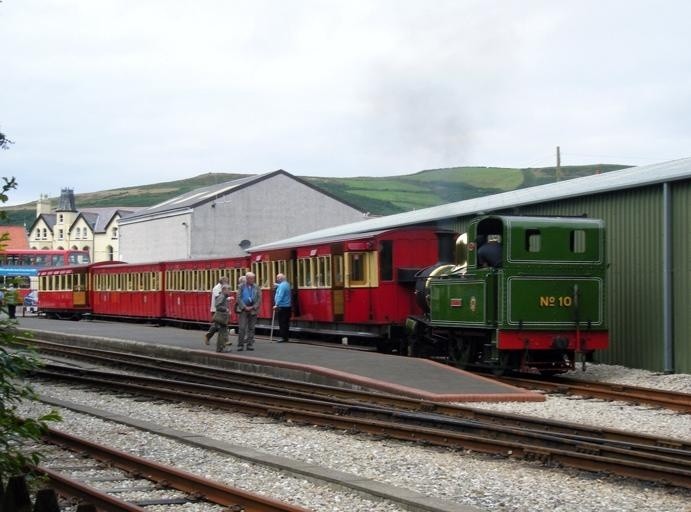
[7,283,18,319]
[205,271,292,352]
[478,233,503,267]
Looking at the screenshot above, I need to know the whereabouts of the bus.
[0,249,91,306]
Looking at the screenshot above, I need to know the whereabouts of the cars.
[23,290,39,314]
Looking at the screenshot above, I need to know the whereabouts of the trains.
[36,215,608,376]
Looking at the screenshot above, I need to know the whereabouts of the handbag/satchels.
[213,311,230,325]
[235,303,243,313]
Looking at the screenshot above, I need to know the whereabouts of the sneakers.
[236,347,244,351]
[203,333,211,345]
[225,341,232,345]
[221,348,231,352]
[247,346,255,351]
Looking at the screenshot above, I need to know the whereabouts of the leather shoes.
[277,339,288,342]
